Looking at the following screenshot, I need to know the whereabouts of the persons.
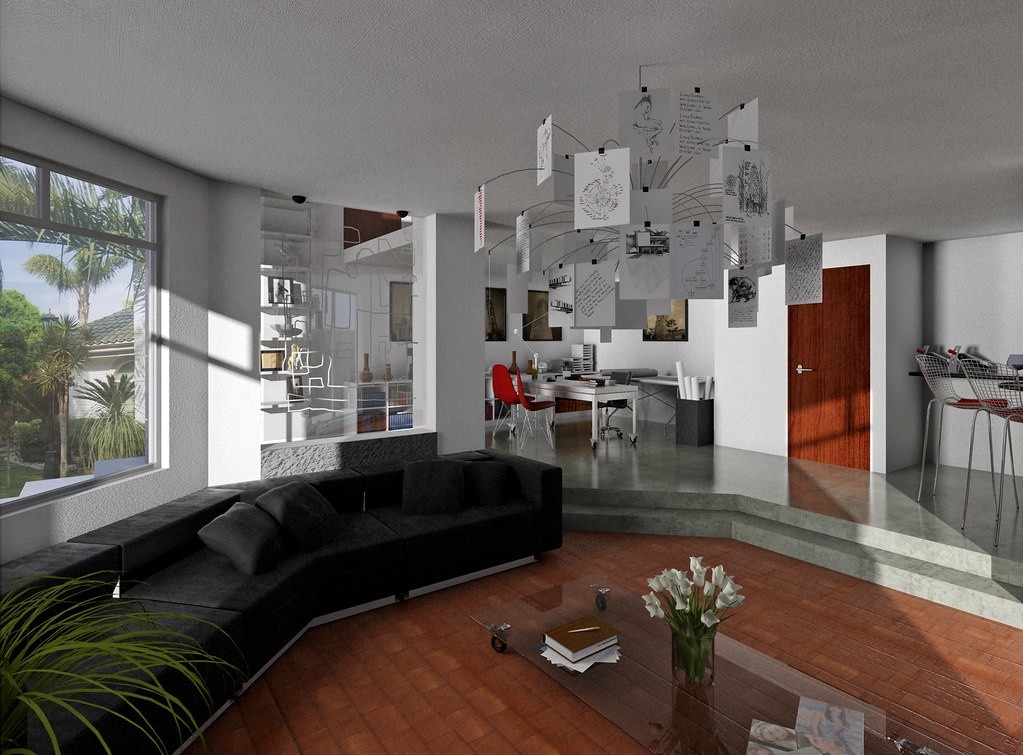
[750,722,795,744]
[811,704,852,755]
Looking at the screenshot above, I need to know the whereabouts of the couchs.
[0,448,563,755]
[1,434,563,755]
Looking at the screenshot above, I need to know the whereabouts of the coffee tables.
[467,574,974,755]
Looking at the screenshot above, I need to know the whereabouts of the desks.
[502,373,639,451]
[581,374,713,437]
[908,371,1023,381]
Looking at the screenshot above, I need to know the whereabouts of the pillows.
[197,501,286,578]
[254,480,342,553]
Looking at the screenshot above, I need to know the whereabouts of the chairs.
[915,354,1021,510]
[492,363,537,450]
[584,371,631,440]
[515,366,555,452]
[956,358,1023,546]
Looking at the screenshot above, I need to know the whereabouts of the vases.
[672,627,714,690]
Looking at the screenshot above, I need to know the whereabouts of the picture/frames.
[484,287,507,341]
[287,376,303,403]
[261,350,287,371]
[521,290,562,341]
[642,299,689,341]
[388,281,412,342]
[268,276,294,304]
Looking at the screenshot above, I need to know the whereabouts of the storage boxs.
[676,399,714,446]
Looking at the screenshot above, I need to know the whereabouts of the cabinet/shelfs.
[342,379,413,435]
[260,197,312,413]
[474,87,827,328]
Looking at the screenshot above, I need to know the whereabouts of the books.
[542,615,619,664]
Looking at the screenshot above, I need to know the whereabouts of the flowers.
[641,556,746,636]
